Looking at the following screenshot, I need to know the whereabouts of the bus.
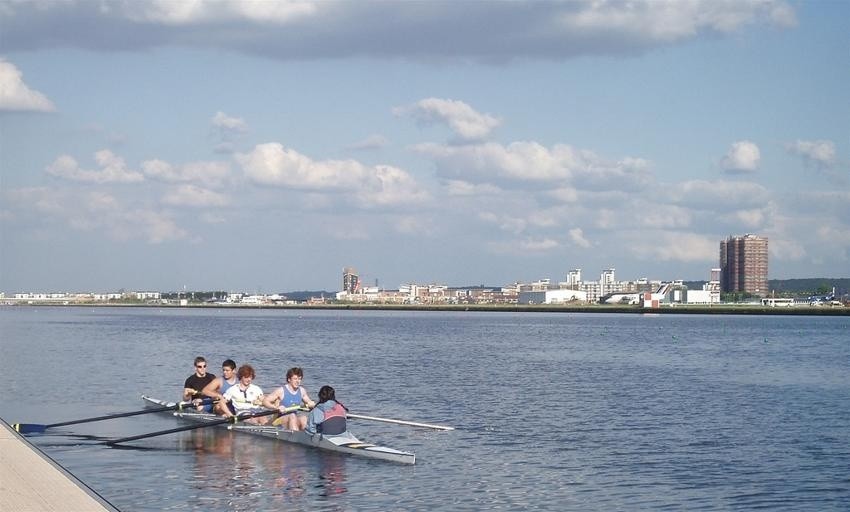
[760,297,796,308]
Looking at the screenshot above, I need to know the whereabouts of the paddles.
[10,397,220,435]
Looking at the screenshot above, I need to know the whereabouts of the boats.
[142,395,415,464]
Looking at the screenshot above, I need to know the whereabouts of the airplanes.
[604,283,672,305]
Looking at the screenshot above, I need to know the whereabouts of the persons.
[218,365,268,426]
[202,359,243,418]
[262,367,315,431]
[305,385,349,437]
[182,356,215,414]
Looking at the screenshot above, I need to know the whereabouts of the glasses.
[196,365,207,368]
